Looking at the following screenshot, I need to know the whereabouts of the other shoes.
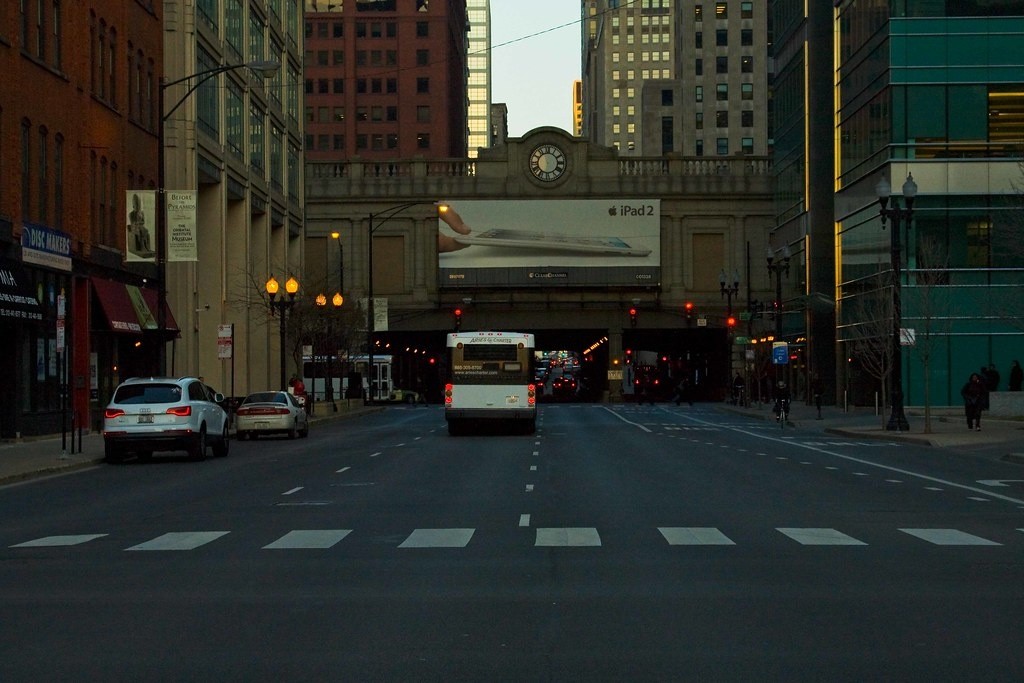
[977,427,981,431]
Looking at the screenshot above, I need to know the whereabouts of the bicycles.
[777,398,787,430]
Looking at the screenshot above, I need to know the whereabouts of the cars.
[392,385,419,404]
[552,358,581,399]
[236,390,308,441]
[536,356,555,397]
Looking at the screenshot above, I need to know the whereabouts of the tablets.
[456,228,653,258]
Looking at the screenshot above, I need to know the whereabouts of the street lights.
[316,291,344,400]
[332,232,343,294]
[266,272,299,391]
[765,241,791,413]
[875,171,918,431]
[156,61,282,377]
[368,199,450,402]
[719,267,740,403]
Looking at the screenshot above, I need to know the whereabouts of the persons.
[128,194,153,252]
[960,373,982,432]
[1008,360,1023,391]
[676,374,693,406]
[198,377,204,382]
[438,205,471,252]
[774,381,790,422]
[289,373,305,396]
[414,376,428,407]
[980,364,999,407]
[734,372,745,406]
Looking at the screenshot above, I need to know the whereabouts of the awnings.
[91,277,182,339]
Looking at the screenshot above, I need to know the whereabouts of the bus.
[302,355,397,405]
[444,332,536,433]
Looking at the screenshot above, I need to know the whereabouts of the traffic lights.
[630,308,636,325]
[727,317,735,327]
[454,308,462,326]
[686,302,692,321]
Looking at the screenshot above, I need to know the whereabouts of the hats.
[776,380,787,388]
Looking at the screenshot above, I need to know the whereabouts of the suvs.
[104,376,230,462]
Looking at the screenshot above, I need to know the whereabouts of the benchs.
[310,399,364,416]
